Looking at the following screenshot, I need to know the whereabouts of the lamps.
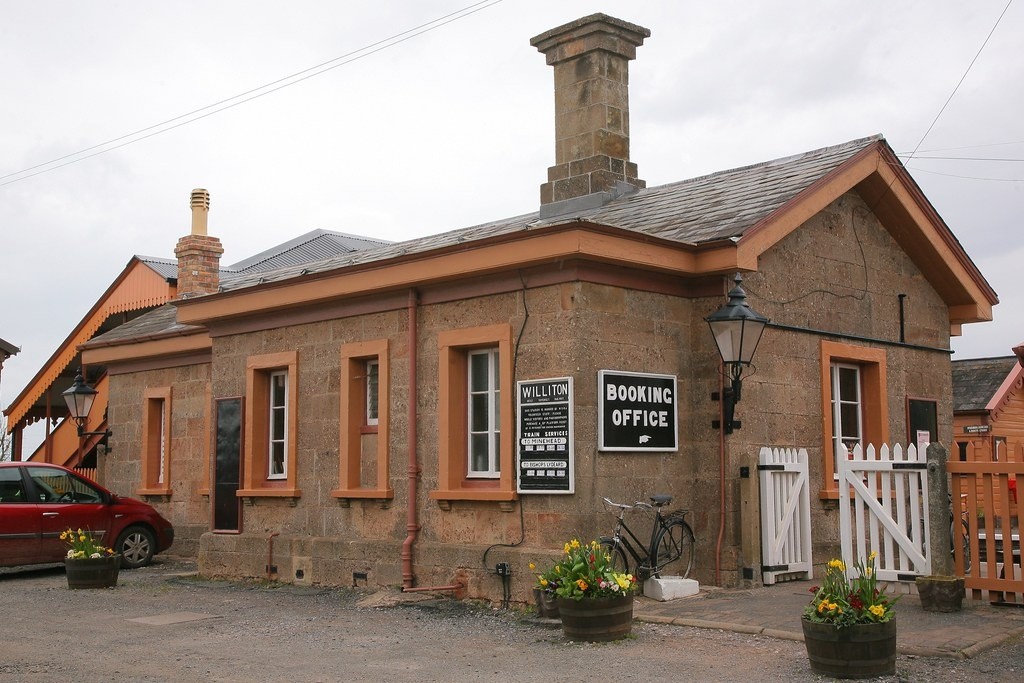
[702,272,770,434]
[61,366,112,455]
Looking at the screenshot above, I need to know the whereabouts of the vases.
[64,557,121,589]
[916,574,965,612]
[801,616,896,678]
[556,596,633,643]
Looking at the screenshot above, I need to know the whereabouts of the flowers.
[58,524,118,558]
[528,528,639,601]
[800,552,904,630]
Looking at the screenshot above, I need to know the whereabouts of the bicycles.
[908,491,972,575]
[588,492,696,583]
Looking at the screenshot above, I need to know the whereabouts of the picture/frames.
[517,376,575,494]
[597,370,679,451]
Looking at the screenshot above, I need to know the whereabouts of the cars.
[0,460,175,569]
